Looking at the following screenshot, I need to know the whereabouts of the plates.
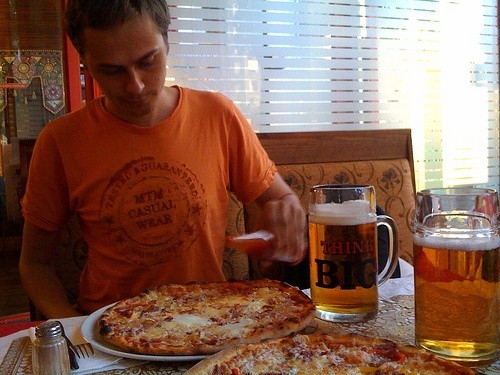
[80,299,218,364]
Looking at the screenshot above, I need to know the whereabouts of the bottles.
[32,323,70,375]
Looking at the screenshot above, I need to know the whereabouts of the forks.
[52,320,94,358]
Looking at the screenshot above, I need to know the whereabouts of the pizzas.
[182,333,473,374]
[94,280,315,354]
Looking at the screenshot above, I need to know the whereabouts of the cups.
[308,182,399,325]
[412,189,496,363]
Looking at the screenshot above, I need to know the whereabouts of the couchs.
[20,129,420,322]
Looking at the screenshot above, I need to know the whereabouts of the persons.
[17,1,306,320]
